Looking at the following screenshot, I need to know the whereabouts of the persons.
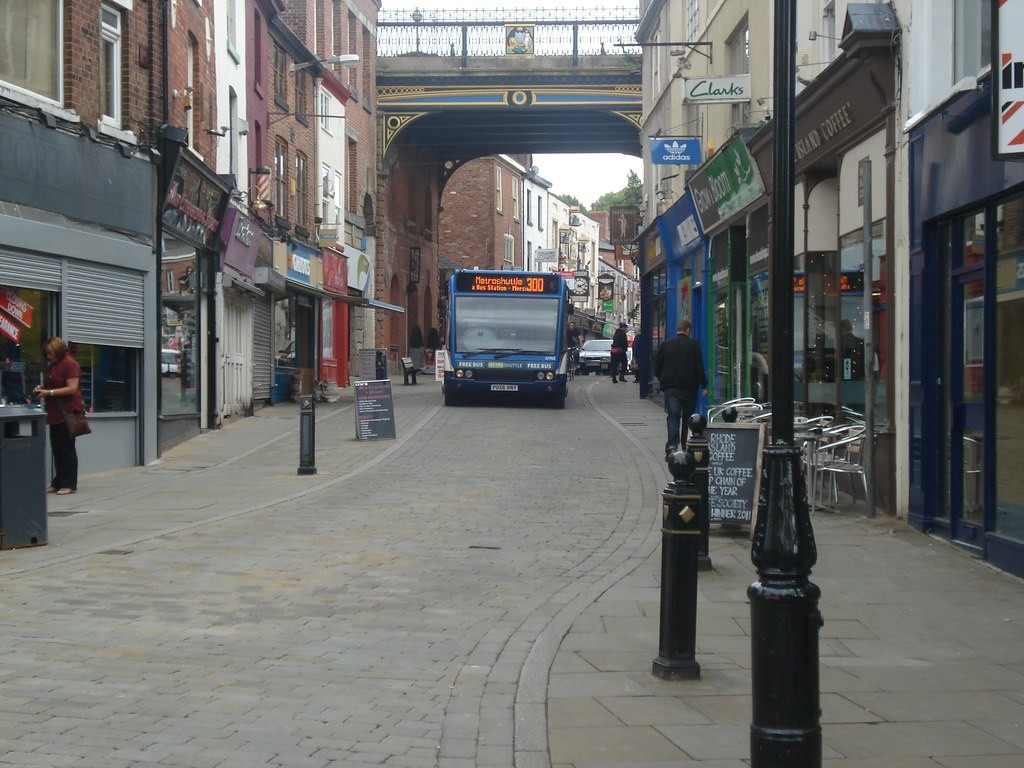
[841,319,865,409]
[567,322,583,373]
[654,319,708,462]
[609,323,629,383]
[33,336,84,494]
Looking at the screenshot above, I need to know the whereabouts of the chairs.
[708,395,872,517]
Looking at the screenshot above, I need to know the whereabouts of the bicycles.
[565,346,578,382]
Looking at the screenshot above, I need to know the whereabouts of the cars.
[622,347,633,374]
[579,339,625,376]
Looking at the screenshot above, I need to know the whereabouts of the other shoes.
[633,378,639,383]
[576,364,580,368]
[56,488,77,495]
[47,486,59,493]
[613,379,618,383]
[665,448,677,462]
[620,377,628,382]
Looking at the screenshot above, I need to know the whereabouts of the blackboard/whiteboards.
[354,379,396,441]
[706,422,766,525]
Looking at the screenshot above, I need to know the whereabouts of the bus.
[441,268,571,410]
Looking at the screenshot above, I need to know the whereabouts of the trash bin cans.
[0,404,49,551]
[270,365,289,404]
